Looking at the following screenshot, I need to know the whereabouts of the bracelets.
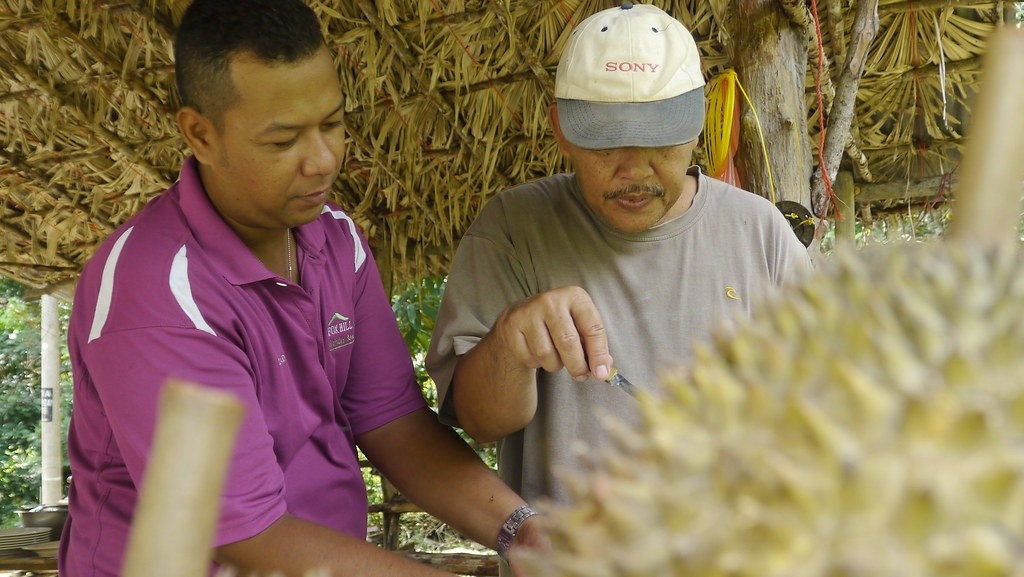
[496,506,538,567]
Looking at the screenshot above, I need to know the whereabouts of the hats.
[555,3,705,149]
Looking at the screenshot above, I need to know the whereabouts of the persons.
[58,0,549,577]
[424,2,815,577]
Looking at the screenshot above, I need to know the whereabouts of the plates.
[0,527,61,558]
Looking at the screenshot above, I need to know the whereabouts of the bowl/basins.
[13,510,68,540]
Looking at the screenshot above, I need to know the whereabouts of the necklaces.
[287,228,292,280]
